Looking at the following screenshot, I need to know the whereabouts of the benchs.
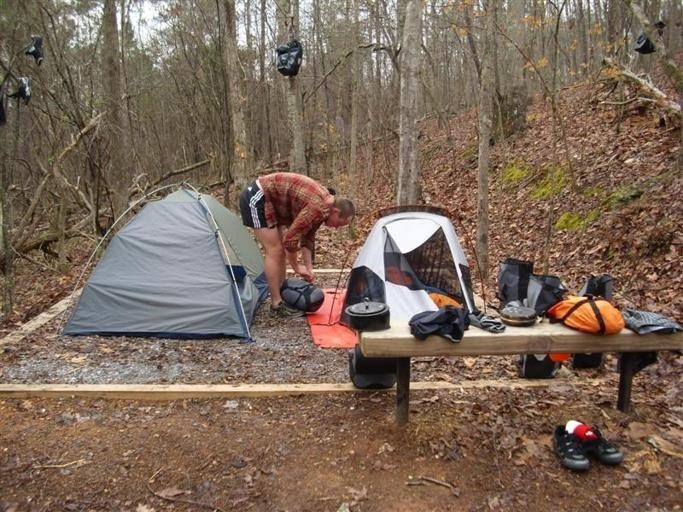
[362,316,682,426]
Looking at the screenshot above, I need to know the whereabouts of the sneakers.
[270,302,303,319]
[583,427,624,463]
[552,426,592,468]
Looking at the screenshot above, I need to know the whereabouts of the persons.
[240,172,355,317]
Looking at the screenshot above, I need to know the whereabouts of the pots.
[346,304,390,329]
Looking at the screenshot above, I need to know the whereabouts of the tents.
[328,204,487,325]
[57,181,269,340]
